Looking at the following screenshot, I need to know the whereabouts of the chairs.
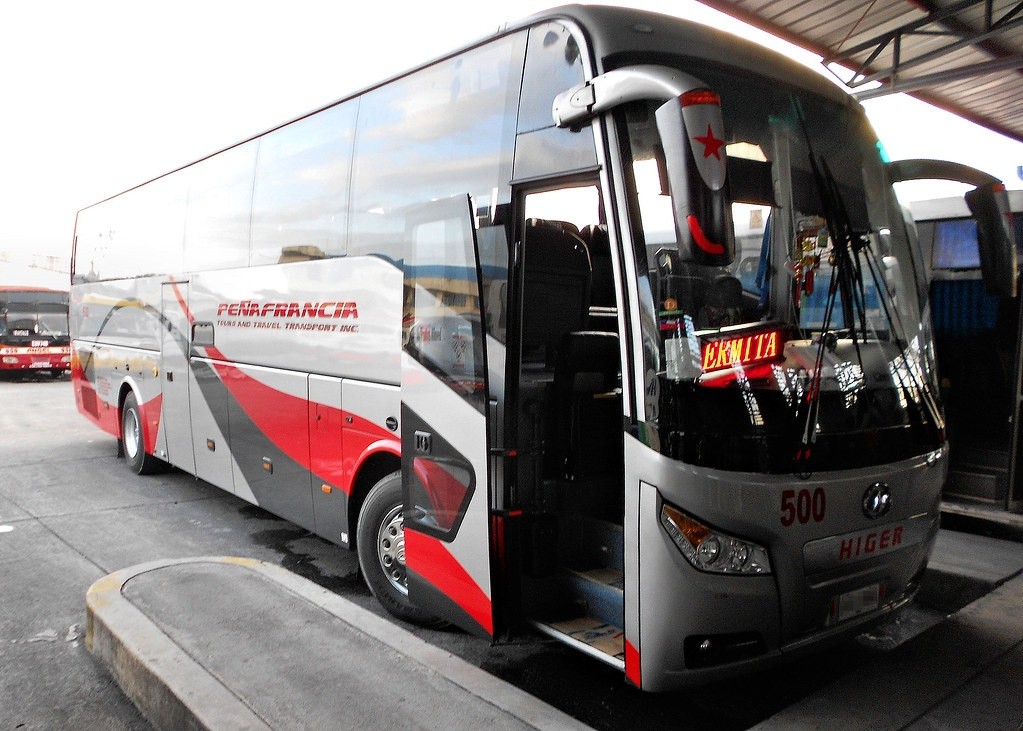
[696,275,744,331]
[652,246,706,329]
[524,224,592,368]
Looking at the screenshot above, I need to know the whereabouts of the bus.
[0,284,73,380]
[72,3,1017,695]
[904,193,1022,536]
